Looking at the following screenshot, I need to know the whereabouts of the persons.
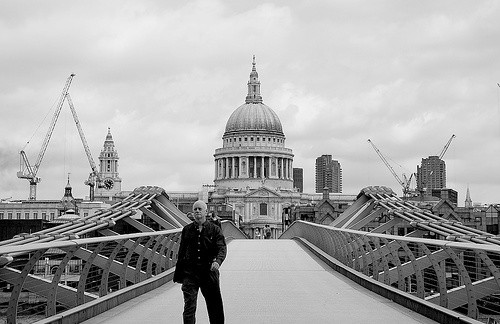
[254,224,272,239]
[172,199,227,324]
[210,211,222,230]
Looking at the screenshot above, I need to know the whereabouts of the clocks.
[103,178,114,190]
